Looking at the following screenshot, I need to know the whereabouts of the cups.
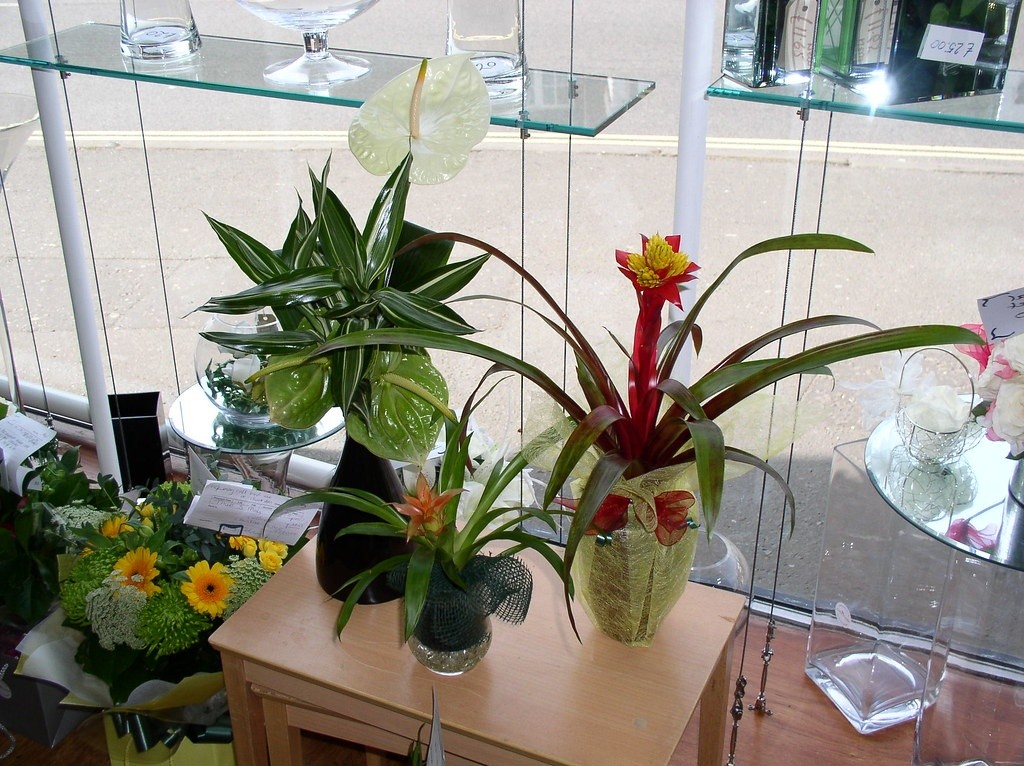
[445,0,533,106]
[118,0,203,69]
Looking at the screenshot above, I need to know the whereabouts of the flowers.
[16,48,983,714]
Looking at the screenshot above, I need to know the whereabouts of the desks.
[167,378,348,503]
[863,390,1024,571]
[206,513,747,766]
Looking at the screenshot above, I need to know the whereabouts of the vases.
[801,438,967,734]
[110,0,529,116]
[193,298,323,433]
[314,431,425,607]
[574,511,699,645]
[403,585,496,676]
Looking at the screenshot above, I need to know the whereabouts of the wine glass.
[236,0,384,88]
